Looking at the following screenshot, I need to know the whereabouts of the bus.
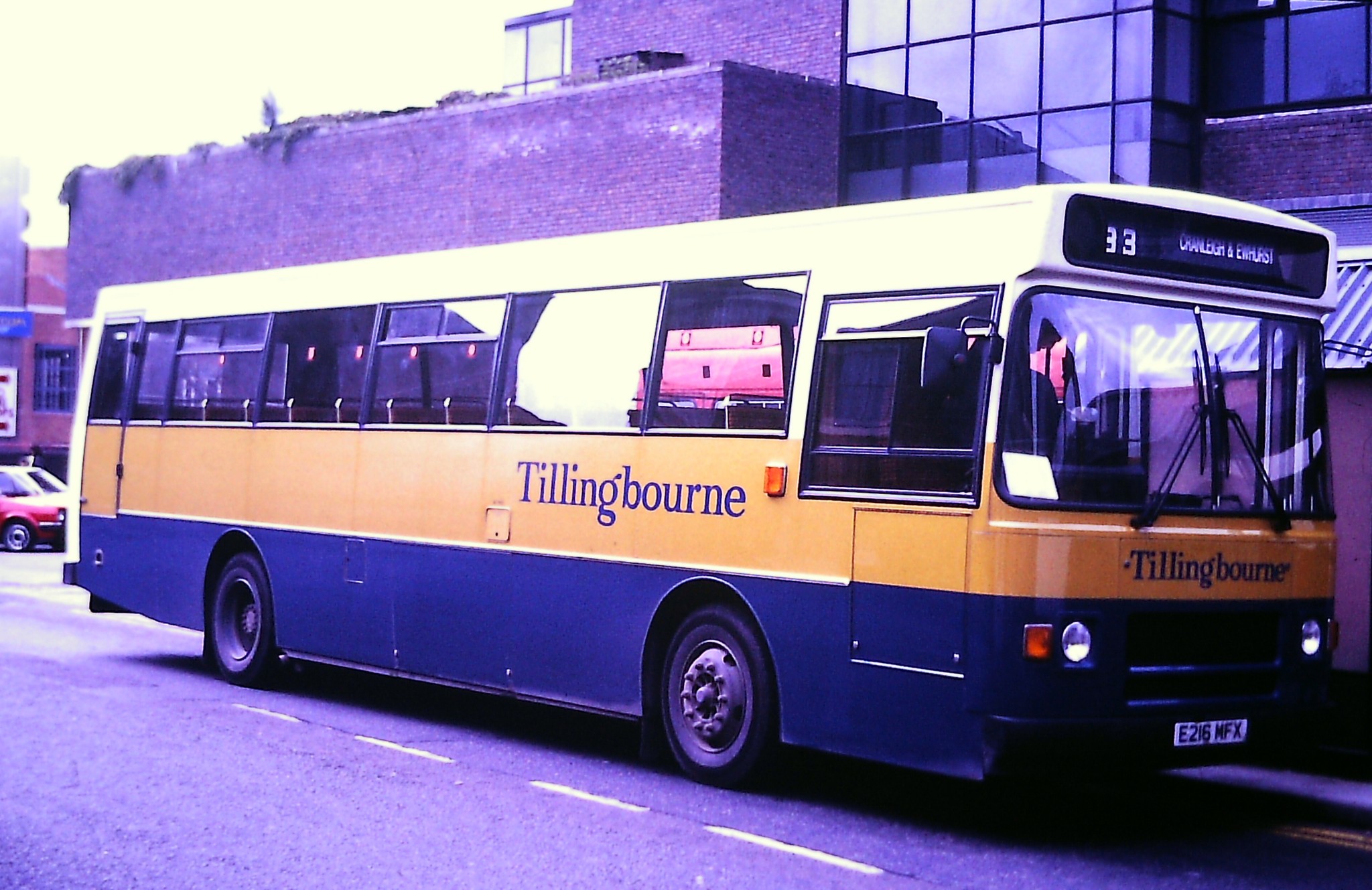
[57,182,1342,788]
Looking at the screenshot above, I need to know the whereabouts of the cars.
[0,469,67,552]
[0,466,70,494]
